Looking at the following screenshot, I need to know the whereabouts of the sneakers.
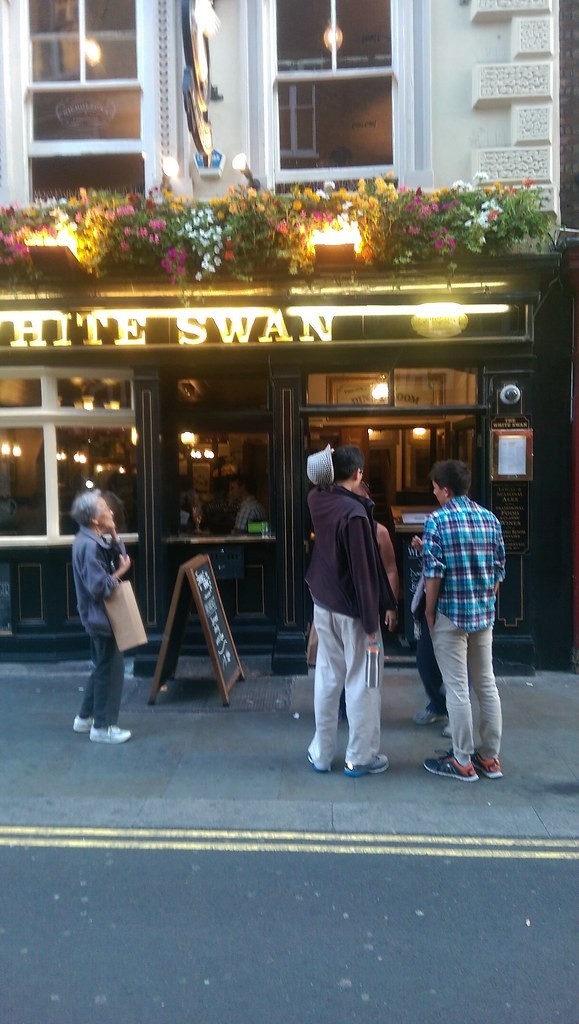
[89,722,132,744]
[471,748,503,779]
[308,751,331,772]
[413,707,448,725]
[345,755,390,778]
[424,748,478,782]
[72,714,94,733]
[442,725,452,739]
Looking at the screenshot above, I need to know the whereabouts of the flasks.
[365,641,380,690]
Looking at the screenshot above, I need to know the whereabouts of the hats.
[307,444,335,486]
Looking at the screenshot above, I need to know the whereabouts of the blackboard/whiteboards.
[150,554,240,696]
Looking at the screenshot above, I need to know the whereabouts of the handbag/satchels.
[101,575,148,652]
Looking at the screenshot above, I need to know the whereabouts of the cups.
[260,521,272,539]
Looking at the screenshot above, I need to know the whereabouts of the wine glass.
[192,506,203,534]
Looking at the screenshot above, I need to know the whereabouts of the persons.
[198,476,267,536]
[68,487,132,744]
[304,447,398,777]
[408,458,508,782]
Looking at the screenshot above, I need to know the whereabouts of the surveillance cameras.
[501,385,521,405]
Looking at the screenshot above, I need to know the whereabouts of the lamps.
[369,374,389,398]
[0,381,215,475]
[309,234,356,277]
[27,243,79,279]
[232,153,260,192]
[159,157,179,194]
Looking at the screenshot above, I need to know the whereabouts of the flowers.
[0,170,558,274]
